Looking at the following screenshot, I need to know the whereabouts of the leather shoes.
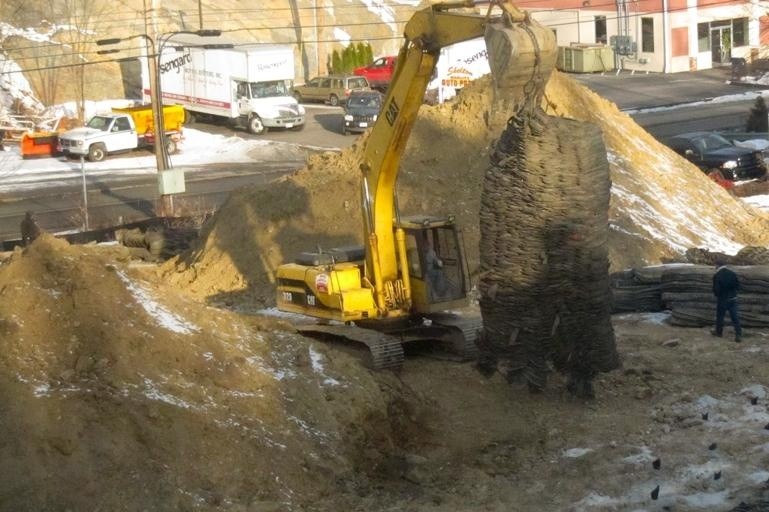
[710,328,723,337]
[735,336,741,342]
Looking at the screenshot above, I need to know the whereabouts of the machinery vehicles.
[256,1,558,373]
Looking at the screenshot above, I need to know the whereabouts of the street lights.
[96,30,222,218]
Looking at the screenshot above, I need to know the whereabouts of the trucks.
[141,33,306,136]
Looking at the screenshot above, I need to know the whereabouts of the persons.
[21,208,41,245]
[710,258,743,342]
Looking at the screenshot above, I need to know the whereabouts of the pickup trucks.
[57,104,185,162]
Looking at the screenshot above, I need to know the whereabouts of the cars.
[293,56,397,136]
[669,132,766,180]
